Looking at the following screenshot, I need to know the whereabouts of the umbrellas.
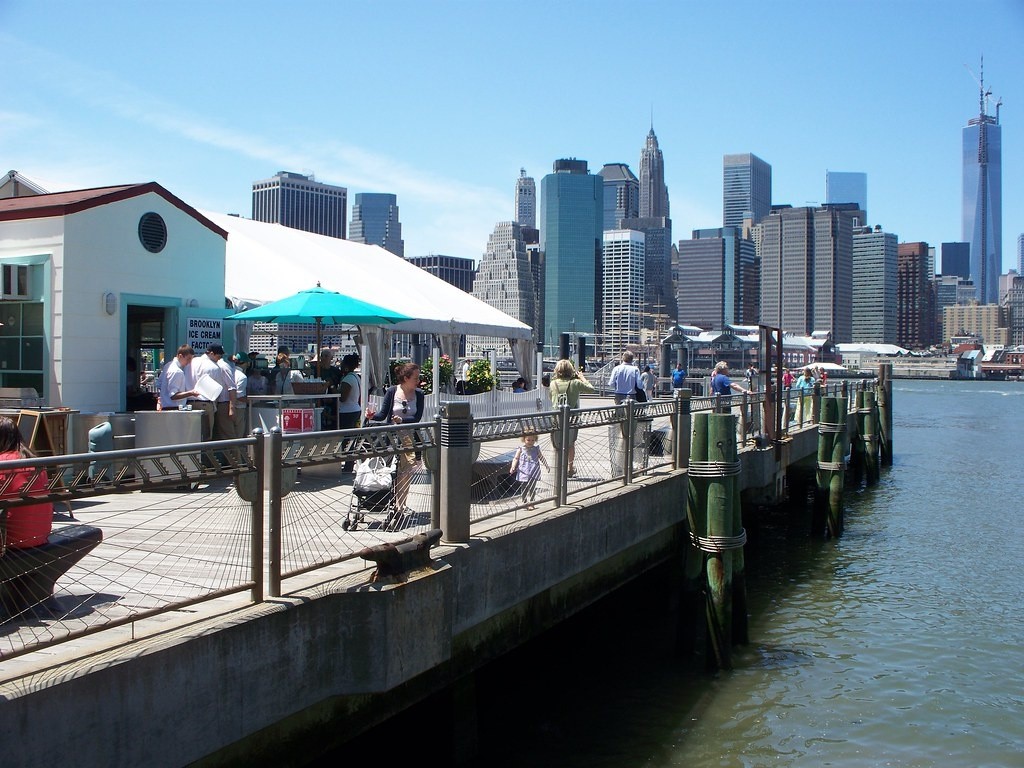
[221,282,416,373]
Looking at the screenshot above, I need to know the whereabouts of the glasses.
[402,401,407,414]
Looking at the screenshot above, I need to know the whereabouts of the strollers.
[343,410,417,530]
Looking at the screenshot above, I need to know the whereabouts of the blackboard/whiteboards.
[16,410,70,503]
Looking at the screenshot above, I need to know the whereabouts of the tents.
[196,207,532,391]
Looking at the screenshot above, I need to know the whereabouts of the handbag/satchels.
[642,431,666,456]
[397,430,418,474]
[635,369,647,402]
[355,457,392,491]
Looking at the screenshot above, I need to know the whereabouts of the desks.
[247,394,341,466]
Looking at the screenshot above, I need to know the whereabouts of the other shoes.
[568,467,577,478]
[399,508,415,515]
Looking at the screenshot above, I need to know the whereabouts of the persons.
[672,363,688,388]
[548,359,595,478]
[744,362,827,428]
[608,350,643,453]
[640,366,657,403]
[463,359,471,381]
[0,415,53,548]
[510,426,550,511]
[140,372,149,392]
[156,343,362,475]
[364,362,424,515]
[511,377,528,393]
[708,360,756,414]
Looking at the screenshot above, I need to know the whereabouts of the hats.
[278,346,288,354]
[237,352,251,363]
[207,343,225,354]
[321,350,335,358]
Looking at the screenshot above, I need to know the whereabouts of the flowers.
[386,353,501,391]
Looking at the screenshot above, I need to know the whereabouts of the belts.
[187,399,212,402]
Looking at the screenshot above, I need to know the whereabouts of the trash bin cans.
[88,422,113,483]
[588,362,601,373]
[600,362,607,368]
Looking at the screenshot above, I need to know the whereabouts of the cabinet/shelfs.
[76,414,136,479]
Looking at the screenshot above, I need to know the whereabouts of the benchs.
[0,523,103,613]
[651,425,671,456]
[472,448,517,498]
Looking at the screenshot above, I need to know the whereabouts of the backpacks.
[555,379,570,405]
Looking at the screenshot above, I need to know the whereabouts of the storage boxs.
[281,408,314,433]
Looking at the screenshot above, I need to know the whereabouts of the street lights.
[770,209,789,475]
